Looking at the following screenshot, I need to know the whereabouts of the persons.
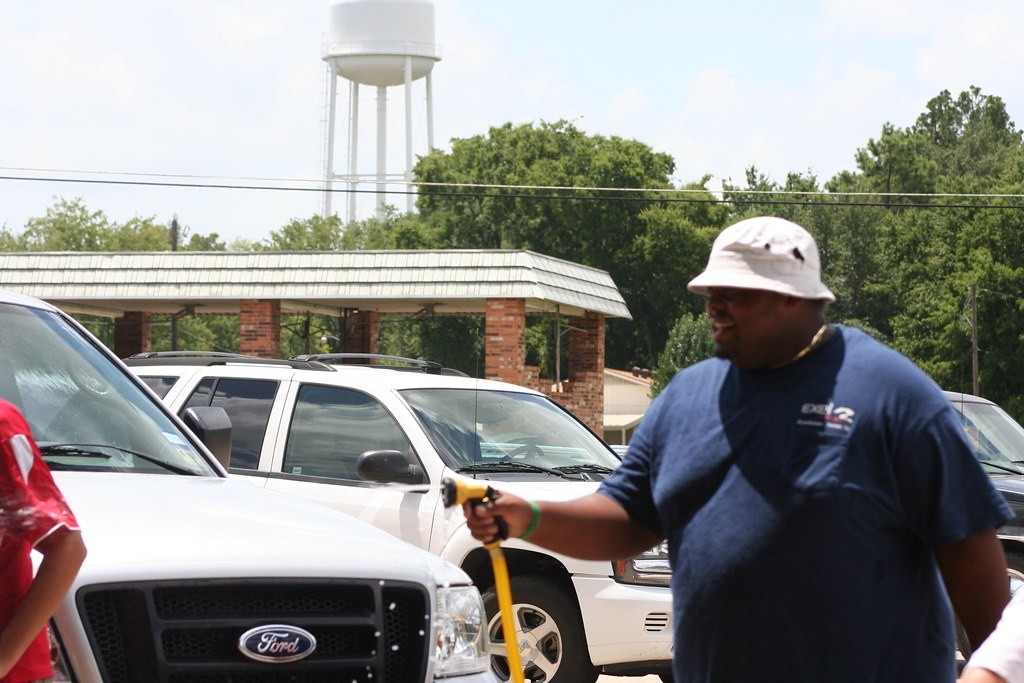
[0,399,87,683]
[459,213,1024,683]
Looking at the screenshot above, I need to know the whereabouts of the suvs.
[120,346,674,683]
[0,288,493,683]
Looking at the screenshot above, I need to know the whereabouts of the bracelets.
[518,502,539,540]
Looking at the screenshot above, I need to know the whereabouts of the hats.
[687,216,835,307]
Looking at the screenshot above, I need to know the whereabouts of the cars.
[943,392,1024,571]
